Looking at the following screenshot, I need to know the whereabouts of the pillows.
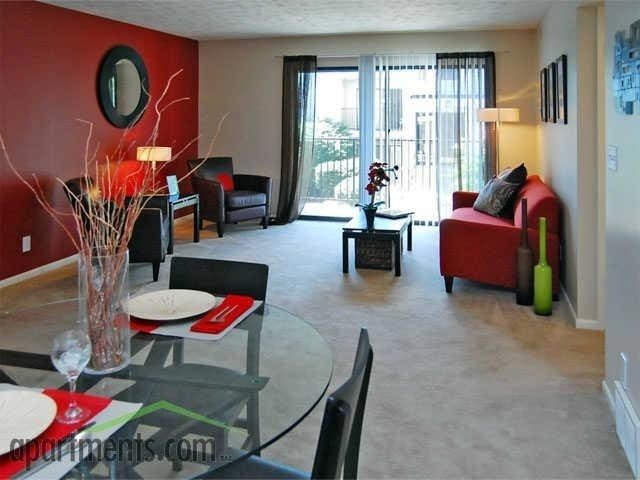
[471,161,528,219]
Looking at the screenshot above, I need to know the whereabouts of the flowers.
[354,160,399,209]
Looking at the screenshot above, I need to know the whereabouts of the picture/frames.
[539,54,568,126]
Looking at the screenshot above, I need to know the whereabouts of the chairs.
[62,174,172,283]
[61,255,375,479]
[186,156,272,238]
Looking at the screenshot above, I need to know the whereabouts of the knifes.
[8,419,97,480]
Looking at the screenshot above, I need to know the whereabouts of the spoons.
[15,430,93,480]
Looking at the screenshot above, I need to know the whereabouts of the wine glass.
[50,329,92,425]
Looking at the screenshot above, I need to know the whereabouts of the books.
[375,207,416,220]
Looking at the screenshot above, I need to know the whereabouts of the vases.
[363,208,379,231]
[515,198,554,317]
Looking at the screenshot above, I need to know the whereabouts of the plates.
[0,389,57,457]
[122,289,217,322]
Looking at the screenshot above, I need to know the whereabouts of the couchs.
[437,173,563,303]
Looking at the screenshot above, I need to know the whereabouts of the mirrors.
[96,44,150,129]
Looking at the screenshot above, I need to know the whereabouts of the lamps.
[136,146,172,193]
[477,107,521,176]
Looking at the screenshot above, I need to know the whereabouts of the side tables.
[166,193,201,254]
